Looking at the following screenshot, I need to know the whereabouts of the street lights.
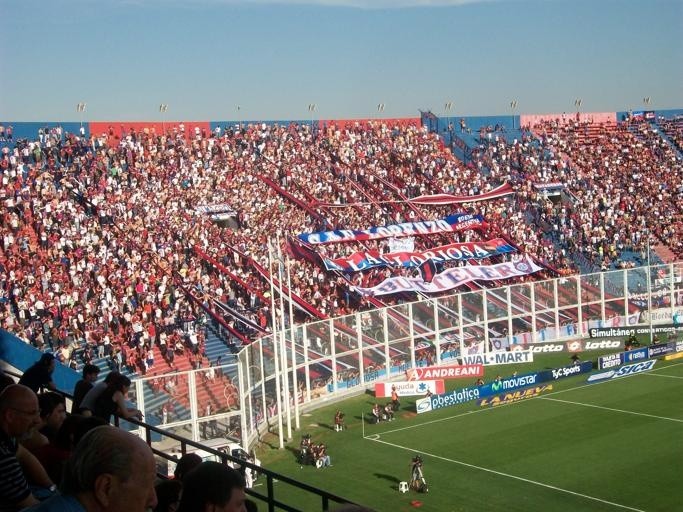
[573,99,583,109]
[643,96,652,106]
[75,101,89,128]
[234,104,243,129]
[510,99,520,129]
[307,103,318,131]
[377,102,386,125]
[158,103,169,135]
[444,102,453,125]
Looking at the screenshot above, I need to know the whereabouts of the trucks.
[148,437,266,490]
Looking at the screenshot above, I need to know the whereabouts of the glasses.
[11,406,41,418]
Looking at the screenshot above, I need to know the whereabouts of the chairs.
[332,118,683,370]
[6,120,332,438]
